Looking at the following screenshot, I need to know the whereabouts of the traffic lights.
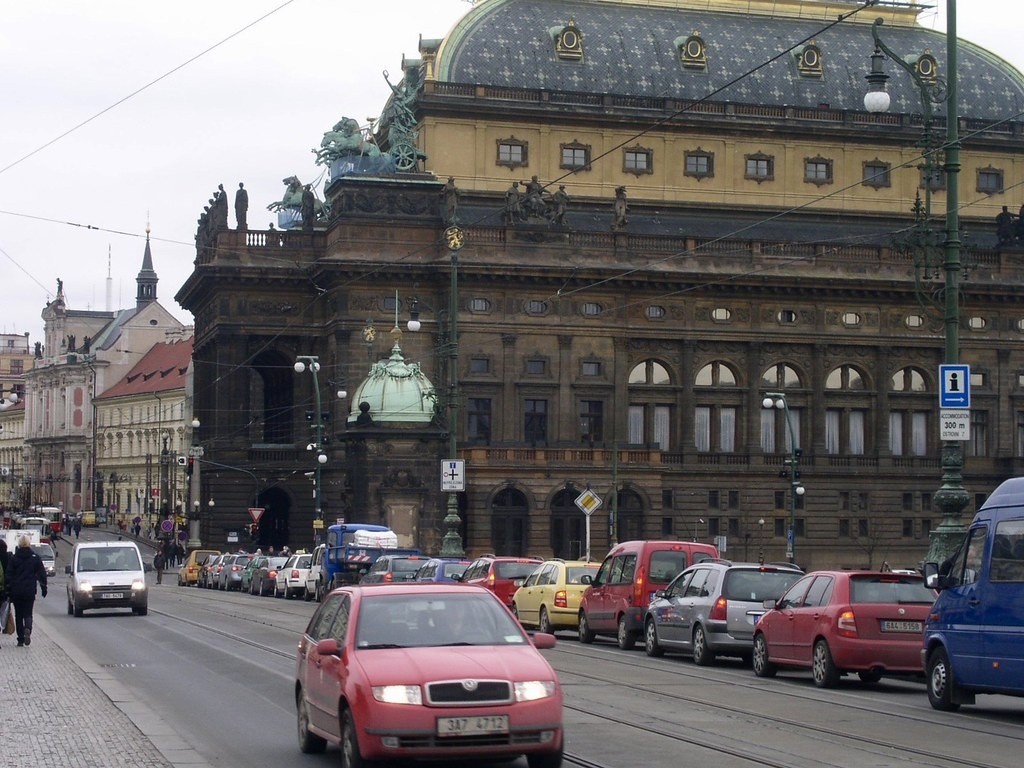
[188,457,194,475]
[178,456,186,466]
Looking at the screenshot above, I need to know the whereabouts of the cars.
[63,513,74,522]
[750,569,939,689]
[405,556,474,582]
[358,554,433,585]
[197,544,325,602]
[452,553,544,611]
[64,541,149,617]
[82,511,99,528]
[510,556,627,637]
[96,517,106,523]
[30,542,58,577]
[177,550,223,587]
[292,581,565,768]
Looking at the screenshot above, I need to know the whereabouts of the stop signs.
[135,517,140,524]
[179,531,186,541]
[111,504,117,510]
[161,520,173,532]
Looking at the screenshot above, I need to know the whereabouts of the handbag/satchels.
[0,600,15,635]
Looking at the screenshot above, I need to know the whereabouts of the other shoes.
[24,627,30,645]
[156,582,161,584]
[17,641,23,646]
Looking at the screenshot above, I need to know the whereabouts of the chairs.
[81,558,96,569]
[363,606,399,643]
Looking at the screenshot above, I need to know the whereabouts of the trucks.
[0,530,41,554]
[319,523,421,603]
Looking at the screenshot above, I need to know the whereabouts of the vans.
[578,540,719,650]
[921,476,1023,713]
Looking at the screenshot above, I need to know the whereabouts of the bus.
[29,504,63,533]
[10,510,51,545]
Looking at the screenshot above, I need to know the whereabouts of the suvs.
[642,557,808,668]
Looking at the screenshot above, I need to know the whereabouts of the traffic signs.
[939,365,971,410]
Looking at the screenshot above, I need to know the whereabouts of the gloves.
[41,586,47,598]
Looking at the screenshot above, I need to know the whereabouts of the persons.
[154,549,165,584]
[158,539,184,570]
[6,535,47,646]
[255,548,263,556]
[73,520,81,539]
[267,545,275,556]
[430,600,488,643]
[0,539,14,648]
[47,527,56,548]
[117,520,125,534]
[278,545,293,557]
[135,523,140,538]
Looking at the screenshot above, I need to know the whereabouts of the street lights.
[1,388,18,403]
[293,354,327,548]
[0,429,14,434]
[864,42,972,570]
[176,416,215,549]
[761,390,805,559]
[407,298,467,557]
[146,432,178,541]
